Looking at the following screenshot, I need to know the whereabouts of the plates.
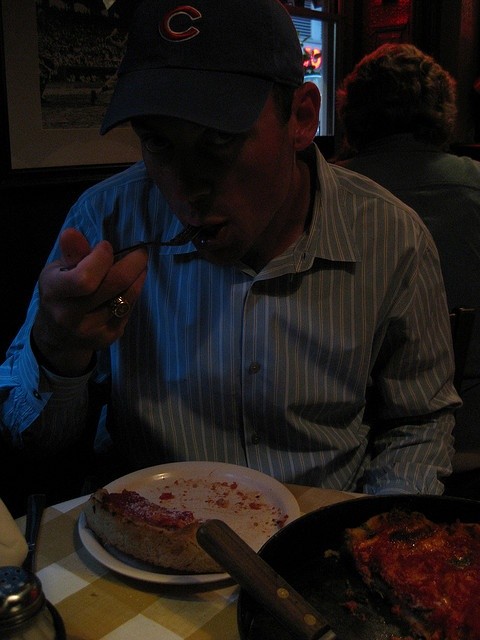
[77,460,300,585]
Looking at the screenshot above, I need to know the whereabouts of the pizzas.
[345,511,479,640]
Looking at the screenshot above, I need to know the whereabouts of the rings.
[110,295,132,319]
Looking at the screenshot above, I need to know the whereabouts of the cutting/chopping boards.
[237,495,480,640]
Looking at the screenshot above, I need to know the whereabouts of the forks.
[60,224,197,273]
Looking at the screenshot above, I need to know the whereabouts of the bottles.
[0,567,57,635]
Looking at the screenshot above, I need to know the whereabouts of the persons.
[0,1,461,497]
[327,42,479,473]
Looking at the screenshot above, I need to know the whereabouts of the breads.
[84,486,221,573]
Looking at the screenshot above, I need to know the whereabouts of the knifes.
[22,491,47,573]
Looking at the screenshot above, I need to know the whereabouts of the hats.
[99,1,304,137]
